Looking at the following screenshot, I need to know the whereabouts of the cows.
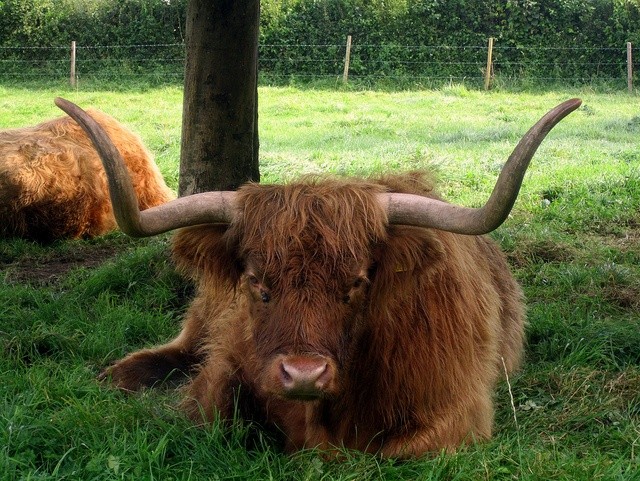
[0,105,180,246]
[51,93,587,468]
